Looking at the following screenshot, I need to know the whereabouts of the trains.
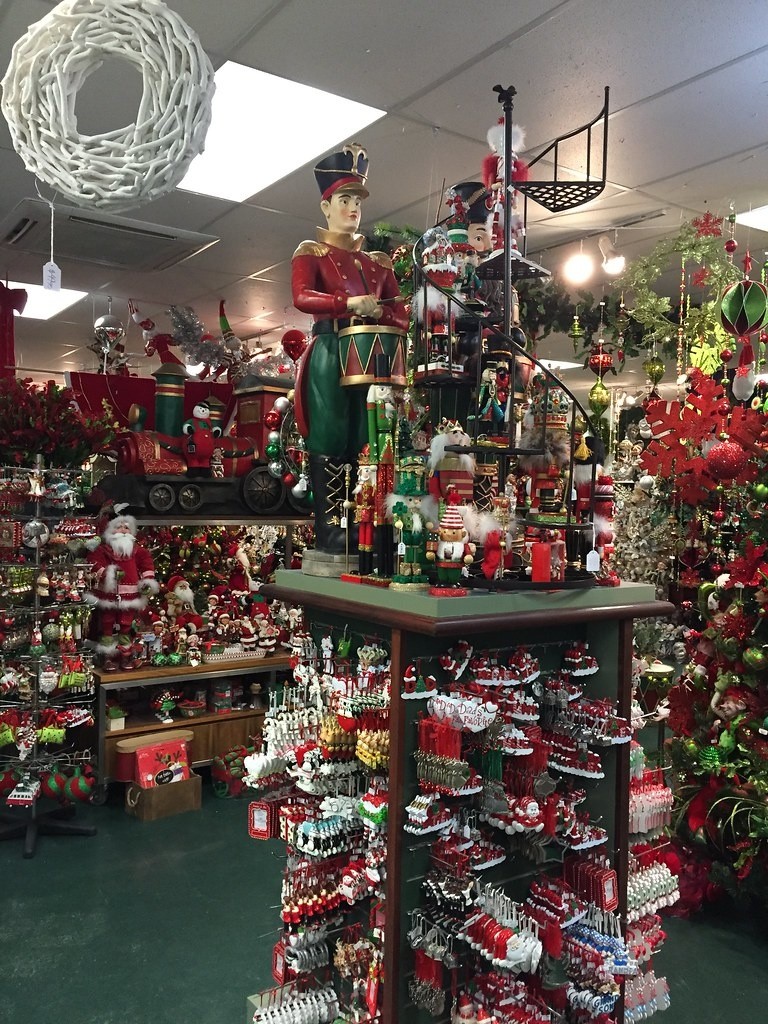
[90,374,316,516]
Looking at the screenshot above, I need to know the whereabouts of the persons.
[0,120,768,1024]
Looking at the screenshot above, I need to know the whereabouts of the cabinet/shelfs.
[89,514,315,806]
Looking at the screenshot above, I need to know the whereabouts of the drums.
[337,325,408,391]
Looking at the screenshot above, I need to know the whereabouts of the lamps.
[598,230,625,275]
